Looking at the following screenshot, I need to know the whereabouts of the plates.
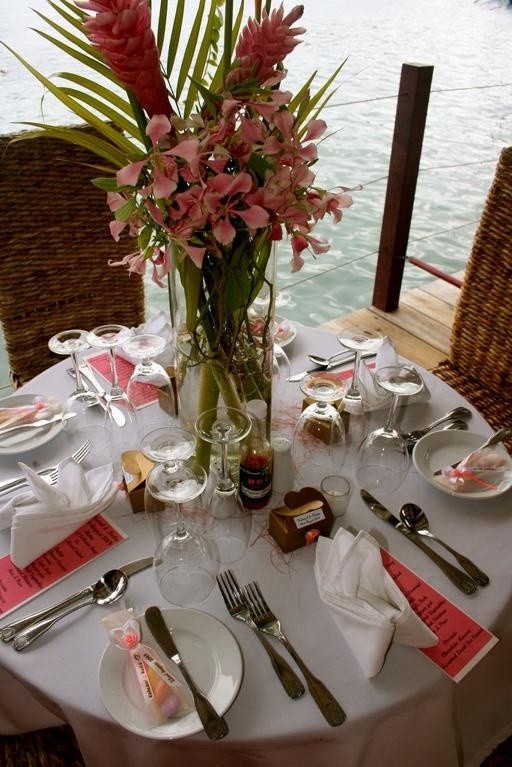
[97,606,245,743]
[413,429,511,499]
[1,394,66,455]
[276,314,295,349]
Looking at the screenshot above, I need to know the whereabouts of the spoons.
[13,567,129,651]
[399,500,490,586]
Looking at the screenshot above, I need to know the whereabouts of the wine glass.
[195,406,251,564]
[146,456,220,606]
[140,426,205,548]
[48,329,112,457]
[291,326,425,495]
[120,332,174,439]
[252,292,292,404]
[86,325,138,457]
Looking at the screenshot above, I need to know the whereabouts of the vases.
[158,226,278,497]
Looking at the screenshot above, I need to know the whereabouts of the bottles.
[239,396,271,507]
[267,435,295,496]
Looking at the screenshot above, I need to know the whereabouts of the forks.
[0,439,92,490]
[402,406,472,455]
[0,464,58,498]
[237,579,347,728]
[215,569,306,700]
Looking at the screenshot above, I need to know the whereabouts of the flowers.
[0,0,355,491]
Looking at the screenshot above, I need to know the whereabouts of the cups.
[320,477,352,517]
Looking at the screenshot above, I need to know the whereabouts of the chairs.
[423,143,512,461]
[2,123,149,393]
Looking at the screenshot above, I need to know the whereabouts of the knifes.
[0,556,162,643]
[359,489,478,597]
[145,607,224,742]
[285,351,377,383]
[1,410,76,435]
[432,429,507,476]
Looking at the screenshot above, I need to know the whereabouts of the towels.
[111,308,182,374]
[1,449,124,575]
[344,336,433,414]
[313,526,442,684]
